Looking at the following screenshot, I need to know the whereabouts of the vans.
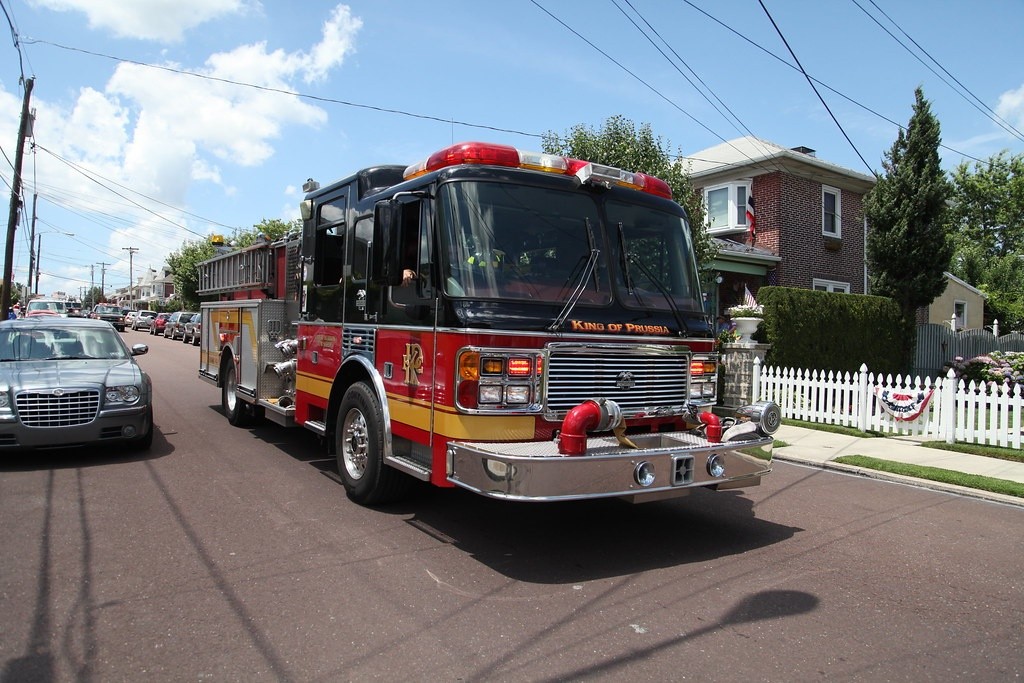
[22,298,58,318]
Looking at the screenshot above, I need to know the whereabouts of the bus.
[61,301,82,318]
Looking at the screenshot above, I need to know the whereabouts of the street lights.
[28,231,74,301]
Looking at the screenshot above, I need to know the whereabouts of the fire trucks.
[194,141,782,502]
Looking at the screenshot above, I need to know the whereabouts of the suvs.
[182,312,201,346]
[131,310,158,331]
[90,303,125,332]
[163,312,197,339]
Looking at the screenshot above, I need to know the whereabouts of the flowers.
[728,305,764,318]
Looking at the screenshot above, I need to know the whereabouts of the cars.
[121,310,136,320]
[150,313,173,336]
[0,319,152,456]
[125,311,138,326]
[22,310,64,339]
